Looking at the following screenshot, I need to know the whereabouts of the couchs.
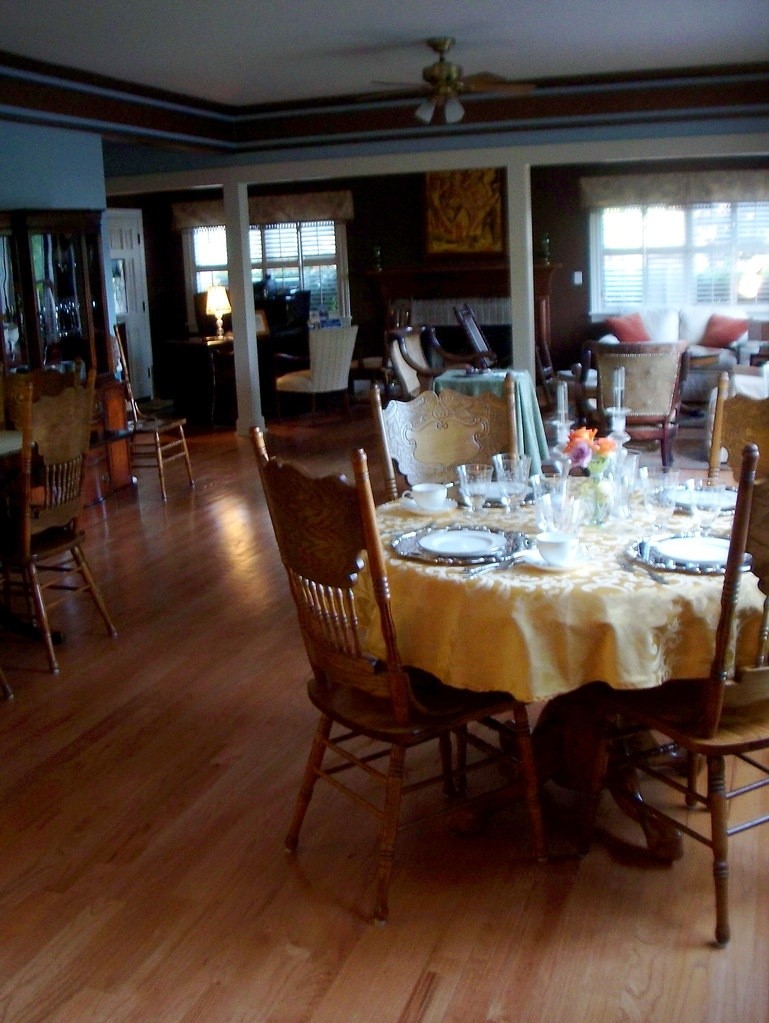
[593,305,769,408]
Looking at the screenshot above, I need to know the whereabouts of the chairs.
[0,327,195,700]
[249,300,769,945]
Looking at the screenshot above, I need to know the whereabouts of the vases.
[570,470,612,527]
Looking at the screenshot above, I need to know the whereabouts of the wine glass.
[492,454,531,524]
[457,464,494,519]
[687,478,727,537]
[530,449,680,539]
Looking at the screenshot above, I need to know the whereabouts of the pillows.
[701,311,748,351]
[607,312,651,344]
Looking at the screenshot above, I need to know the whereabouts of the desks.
[432,369,551,475]
[170,328,305,430]
[330,475,769,867]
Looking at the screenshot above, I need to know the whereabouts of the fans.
[370,34,537,98]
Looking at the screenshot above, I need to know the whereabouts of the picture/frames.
[421,167,507,261]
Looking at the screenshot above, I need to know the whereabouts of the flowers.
[562,426,616,468]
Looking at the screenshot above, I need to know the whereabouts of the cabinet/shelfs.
[0,206,132,508]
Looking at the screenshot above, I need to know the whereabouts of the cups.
[402,483,447,508]
[537,534,582,565]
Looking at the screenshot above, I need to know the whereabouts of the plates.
[669,490,740,509]
[403,499,458,516]
[523,553,591,572]
[420,532,507,557]
[657,537,731,563]
[460,483,530,501]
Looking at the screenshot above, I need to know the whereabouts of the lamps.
[413,94,466,126]
[203,286,232,344]
